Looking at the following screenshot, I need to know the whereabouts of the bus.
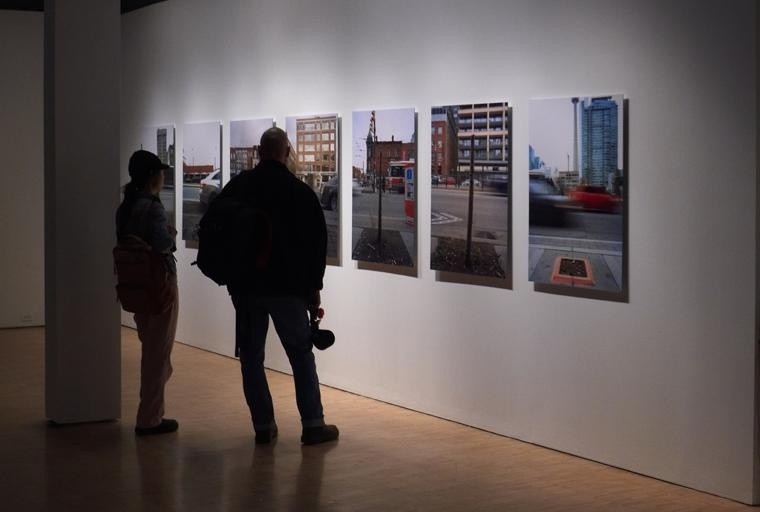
[387,159,415,195]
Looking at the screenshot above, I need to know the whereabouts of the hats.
[129,150,170,176]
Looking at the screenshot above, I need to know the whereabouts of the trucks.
[183,163,213,183]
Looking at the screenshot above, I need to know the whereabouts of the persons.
[114,150,178,436]
[197,128,337,447]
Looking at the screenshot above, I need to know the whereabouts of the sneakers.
[136,420,178,436]
[301,425,338,444]
[255,424,278,444]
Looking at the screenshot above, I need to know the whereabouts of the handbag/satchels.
[113,235,161,313]
[197,175,238,286]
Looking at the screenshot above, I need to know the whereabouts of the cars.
[440,176,456,186]
[432,176,439,185]
[569,185,623,213]
[199,169,221,208]
[320,175,338,212]
[462,179,482,188]
[529,172,571,224]
